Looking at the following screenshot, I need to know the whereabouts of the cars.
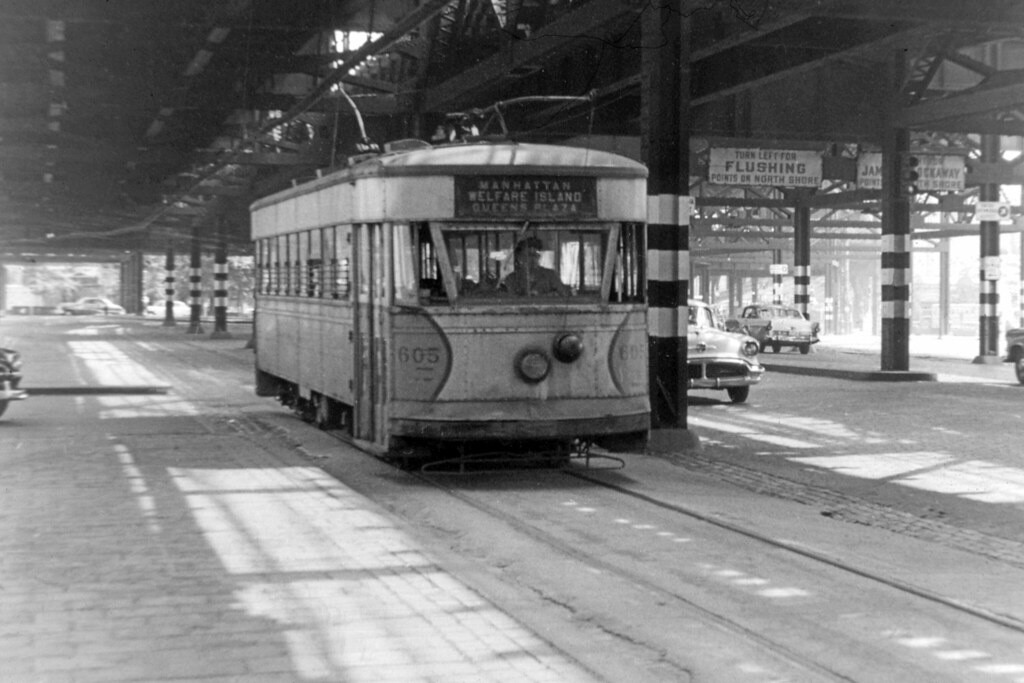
[725,304,819,354]
[686,299,765,402]
[57,298,193,319]
[1003,329,1024,385]
[0,348,28,420]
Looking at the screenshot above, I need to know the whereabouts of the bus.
[241,82,651,476]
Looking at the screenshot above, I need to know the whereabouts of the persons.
[506,237,573,297]
